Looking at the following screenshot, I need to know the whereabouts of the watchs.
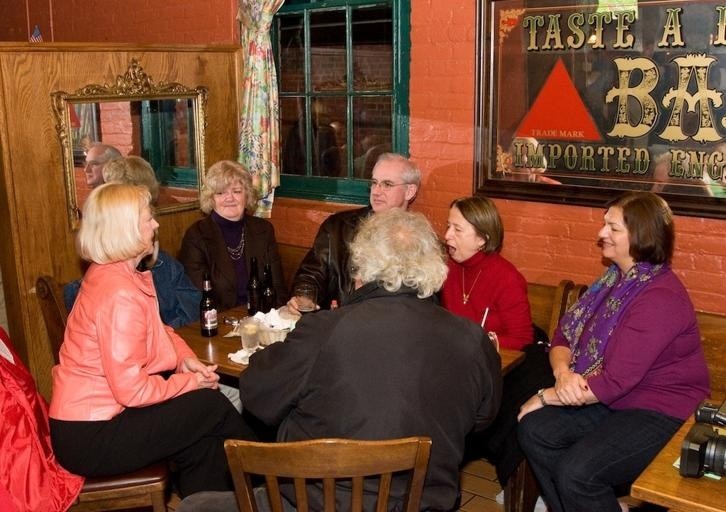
[536,387,548,408]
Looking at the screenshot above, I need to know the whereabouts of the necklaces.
[460,267,483,311]
[226,228,247,263]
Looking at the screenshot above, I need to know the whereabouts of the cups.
[293,285,320,314]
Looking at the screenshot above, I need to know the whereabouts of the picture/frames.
[472,0,726,219]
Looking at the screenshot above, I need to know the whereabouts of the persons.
[284,116,387,177]
[47,180,247,511]
[438,195,544,511]
[287,152,420,318]
[83,143,122,189]
[501,136,562,185]
[180,159,287,317]
[494,190,712,512]
[176,208,504,512]
[648,150,718,195]
[63,204,204,333]
[103,155,159,213]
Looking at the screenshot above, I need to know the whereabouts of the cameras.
[695,398,726,427]
[680,423,726,478]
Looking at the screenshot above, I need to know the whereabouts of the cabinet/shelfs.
[0,41,241,403]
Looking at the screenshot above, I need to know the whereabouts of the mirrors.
[50,57,208,231]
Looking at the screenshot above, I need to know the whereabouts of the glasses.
[368,177,417,189]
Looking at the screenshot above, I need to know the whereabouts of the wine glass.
[239,319,260,364]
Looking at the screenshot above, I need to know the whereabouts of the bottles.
[244,253,277,314]
[198,269,218,337]
